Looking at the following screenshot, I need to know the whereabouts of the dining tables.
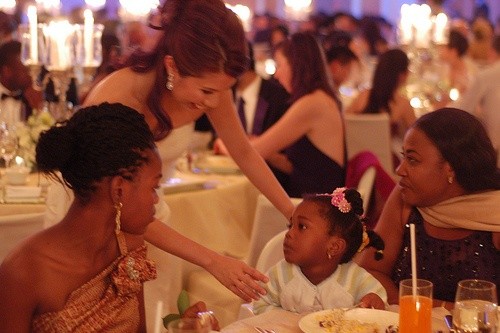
[0,150,261,333]
[216,305,451,333]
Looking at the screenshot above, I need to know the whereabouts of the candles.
[48,21,69,72]
[27,5,39,62]
[82,7,97,62]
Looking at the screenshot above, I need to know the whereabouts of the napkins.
[5,184,42,203]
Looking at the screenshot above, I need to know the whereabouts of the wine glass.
[0,121,18,168]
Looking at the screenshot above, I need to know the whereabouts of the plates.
[160,154,241,195]
[299,307,399,333]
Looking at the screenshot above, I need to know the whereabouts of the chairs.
[342,113,394,179]
[190,195,305,327]
[235,225,290,320]
[346,148,379,223]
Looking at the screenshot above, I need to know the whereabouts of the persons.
[356,107,500,308]
[1,101,162,333]
[0,0,159,118]
[252,187,391,314]
[194,0,500,198]
[45,0,297,333]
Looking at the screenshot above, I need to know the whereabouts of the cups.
[399,278,433,333]
[168,318,211,333]
[48,103,72,124]
[452,279,500,333]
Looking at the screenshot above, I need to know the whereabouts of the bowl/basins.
[1,166,32,184]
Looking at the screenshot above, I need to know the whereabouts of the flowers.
[332,186,353,213]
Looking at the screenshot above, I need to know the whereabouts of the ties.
[238,98,247,134]
